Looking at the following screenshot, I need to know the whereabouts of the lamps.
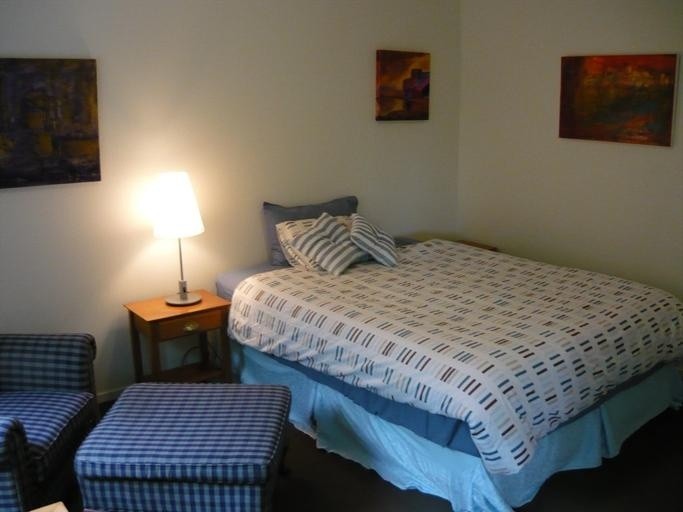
[151,171,205,306]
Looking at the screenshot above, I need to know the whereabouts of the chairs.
[0,334,102,512]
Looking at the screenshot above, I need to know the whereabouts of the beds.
[215,237,683,512]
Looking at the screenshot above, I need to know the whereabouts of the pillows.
[264,196,398,276]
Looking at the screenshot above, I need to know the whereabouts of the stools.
[73,382,292,512]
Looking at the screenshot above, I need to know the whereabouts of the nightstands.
[123,289,233,383]
